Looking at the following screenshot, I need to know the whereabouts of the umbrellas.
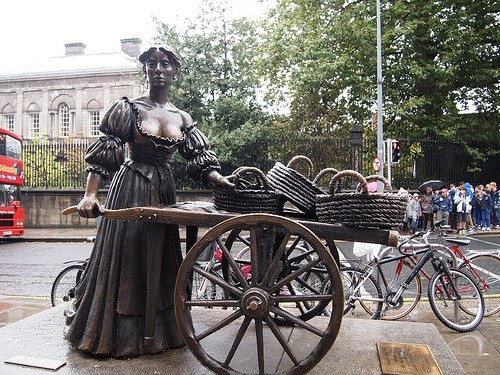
[418,180,443,193]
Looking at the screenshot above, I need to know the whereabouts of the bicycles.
[183,219,500,332]
[51,259,89,308]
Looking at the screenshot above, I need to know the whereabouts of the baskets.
[196,242,217,262]
[212,166,286,214]
[316,170,410,227]
[263,154,343,215]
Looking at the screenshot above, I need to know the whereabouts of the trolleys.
[62,200,400,375]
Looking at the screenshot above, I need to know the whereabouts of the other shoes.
[412,224,500,236]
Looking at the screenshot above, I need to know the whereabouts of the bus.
[0,127,24,236]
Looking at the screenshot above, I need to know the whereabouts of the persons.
[398,181,500,237]
[63,44,236,359]
